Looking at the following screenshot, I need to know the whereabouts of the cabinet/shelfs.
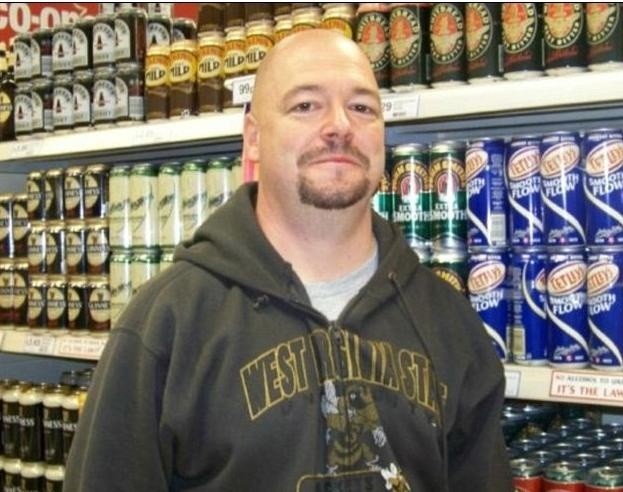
[0,69,623,411]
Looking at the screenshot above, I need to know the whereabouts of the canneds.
[0,2,623,141]
[372,127,621,371]
[498,402,622,491]
[2,365,95,491]
[2,156,243,333]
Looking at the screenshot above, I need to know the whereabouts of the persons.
[63,28,511,492]
